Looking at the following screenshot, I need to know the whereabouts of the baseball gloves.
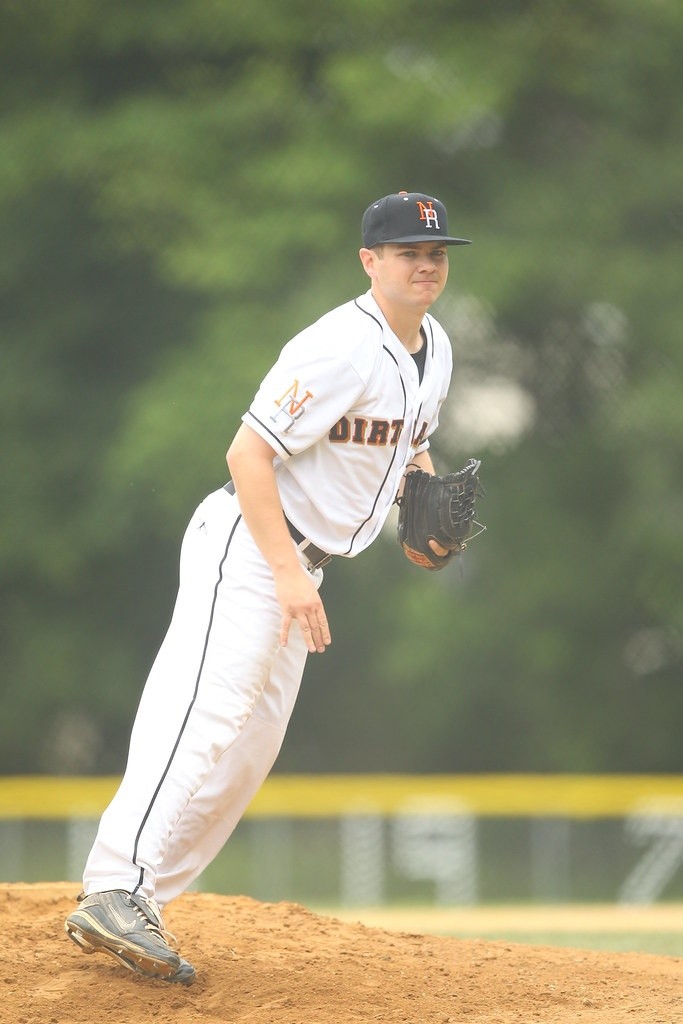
[396,457,483,572]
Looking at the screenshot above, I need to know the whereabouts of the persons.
[64,191,469,989]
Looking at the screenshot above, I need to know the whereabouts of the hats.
[363,190,472,249]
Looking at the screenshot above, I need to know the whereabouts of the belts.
[225,479,334,574]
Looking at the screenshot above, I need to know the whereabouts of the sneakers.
[66,888,181,978]
[64,919,197,987]
